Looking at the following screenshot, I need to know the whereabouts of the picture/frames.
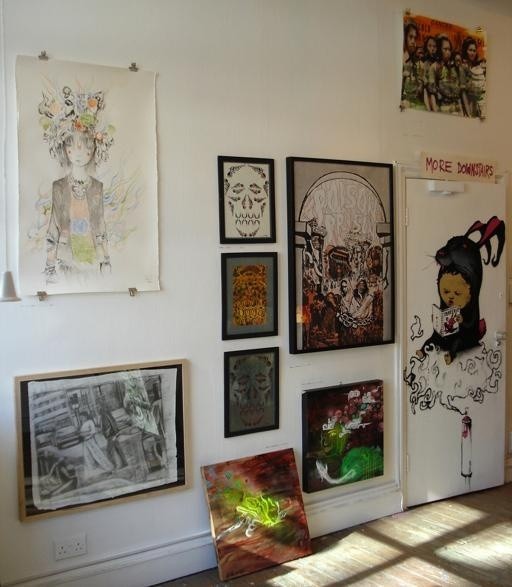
[12,357,195,525]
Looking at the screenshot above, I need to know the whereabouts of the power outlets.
[52,531,89,561]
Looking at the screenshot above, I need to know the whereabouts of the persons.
[79,411,116,485]
[93,398,129,470]
[401,19,485,115]
